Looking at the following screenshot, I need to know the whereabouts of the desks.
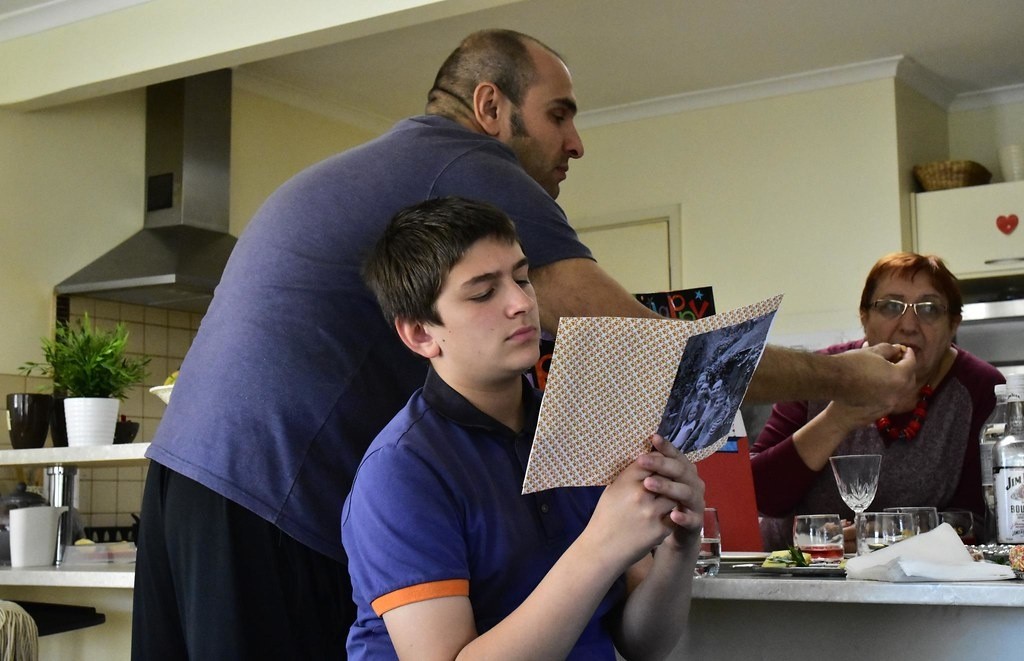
[611,551,1024,661]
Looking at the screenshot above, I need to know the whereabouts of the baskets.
[914,160,991,191]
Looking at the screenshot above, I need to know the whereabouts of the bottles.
[978,371,1024,545]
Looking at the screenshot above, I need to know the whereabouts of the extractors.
[54,65,239,314]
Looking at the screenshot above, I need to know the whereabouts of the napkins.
[843,520,1016,580]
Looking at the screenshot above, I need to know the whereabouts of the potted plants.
[16,309,154,448]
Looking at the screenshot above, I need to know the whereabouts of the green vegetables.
[768,542,809,567]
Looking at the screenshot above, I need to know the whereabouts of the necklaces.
[876,383,932,442]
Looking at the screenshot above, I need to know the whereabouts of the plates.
[759,559,845,577]
[720,550,776,561]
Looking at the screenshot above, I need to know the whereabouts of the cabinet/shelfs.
[0,442,152,661]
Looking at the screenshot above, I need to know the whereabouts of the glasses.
[866,299,949,324]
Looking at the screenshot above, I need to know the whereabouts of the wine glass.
[828,453,883,555]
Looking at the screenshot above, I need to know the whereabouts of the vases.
[6,393,53,450]
[50,396,75,447]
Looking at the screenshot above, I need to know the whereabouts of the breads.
[761,550,811,568]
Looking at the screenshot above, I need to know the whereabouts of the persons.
[131,28,913,661]
[749,253,1006,548]
[339,198,705,661]
[669,372,730,456]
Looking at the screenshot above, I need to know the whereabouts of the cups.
[935,510,974,538]
[691,508,722,578]
[854,506,939,558]
[997,144,1024,181]
[792,514,845,563]
